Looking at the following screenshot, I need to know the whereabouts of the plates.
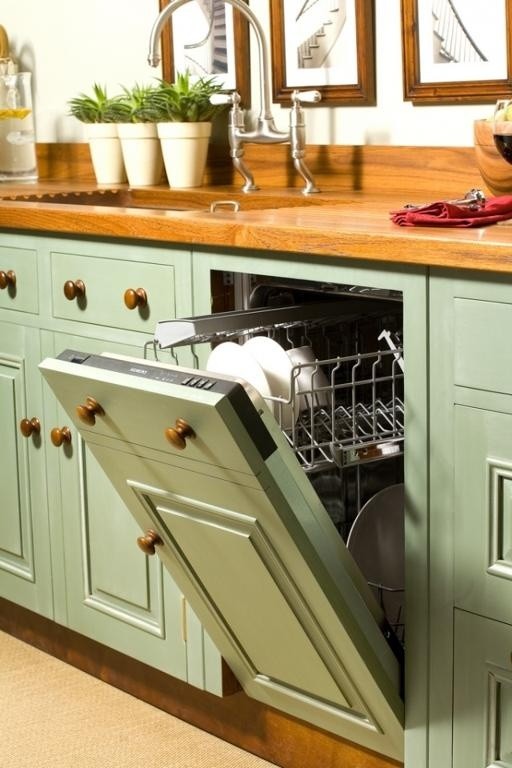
[346,484,405,632]
[204,340,275,419]
[242,336,301,435]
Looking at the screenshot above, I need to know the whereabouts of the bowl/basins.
[472,117,512,204]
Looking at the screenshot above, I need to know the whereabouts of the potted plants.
[67,66,231,191]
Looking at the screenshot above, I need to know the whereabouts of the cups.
[285,346,340,409]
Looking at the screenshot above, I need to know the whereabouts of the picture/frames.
[400,1,511,106]
[158,1,252,111]
[269,0,378,109]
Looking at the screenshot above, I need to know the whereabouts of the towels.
[391,192,512,229]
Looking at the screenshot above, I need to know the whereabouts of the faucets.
[147,0,270,115]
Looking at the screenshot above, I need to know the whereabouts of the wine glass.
[492,120,512,168]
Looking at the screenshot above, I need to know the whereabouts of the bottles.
[0,55,41,187]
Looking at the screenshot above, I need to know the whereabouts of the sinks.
[0,190,350,213]
